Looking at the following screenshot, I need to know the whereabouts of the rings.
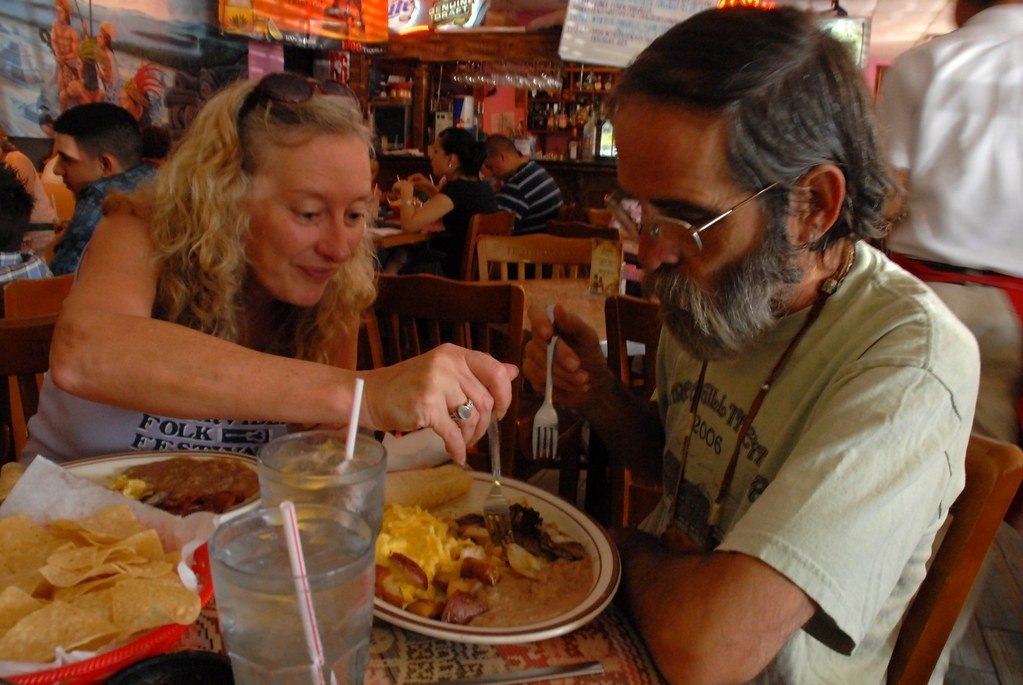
[449,397,474,424]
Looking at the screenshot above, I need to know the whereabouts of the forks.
[483,409,515,549]
[533,303,565,457]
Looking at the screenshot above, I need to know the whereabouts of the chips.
[101,472,156,503]
[0,502,201,661]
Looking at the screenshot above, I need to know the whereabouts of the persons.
[0,102,567,464]
[524,3,983,685]
[873,0,1023,685]
[18,71,521,466]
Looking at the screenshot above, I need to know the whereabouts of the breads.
[358,463,473,513]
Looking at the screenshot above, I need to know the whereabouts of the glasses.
[235,72,362,125]
[603,162,849,256]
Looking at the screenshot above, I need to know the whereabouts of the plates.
[0,491,213,685]
[371,468,622,644]
[54,450,266,523]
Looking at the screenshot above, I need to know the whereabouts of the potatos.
[373,551,500,623]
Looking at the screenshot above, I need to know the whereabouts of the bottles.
[318,0,365,54]
[591,273,604,293]
[224,0,253,33]
[388,135,402,151]
[600,117,615,157]
[576,67,613,91]
[51,216,64,236]
[567,128,580,161]
[527,94,613,131]
[582,110,596,162]
[379,81,386,96]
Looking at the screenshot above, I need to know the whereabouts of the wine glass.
[449,56,563,90]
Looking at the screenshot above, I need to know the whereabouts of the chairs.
[0,204,1023,685]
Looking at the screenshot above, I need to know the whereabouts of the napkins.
[0,454,221,678]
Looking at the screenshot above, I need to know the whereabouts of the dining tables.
[0,449,659,685]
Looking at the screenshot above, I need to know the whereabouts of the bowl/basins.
[389,199,422,216]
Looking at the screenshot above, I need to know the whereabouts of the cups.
[256,428,388,541]
[210,503,374,685]
